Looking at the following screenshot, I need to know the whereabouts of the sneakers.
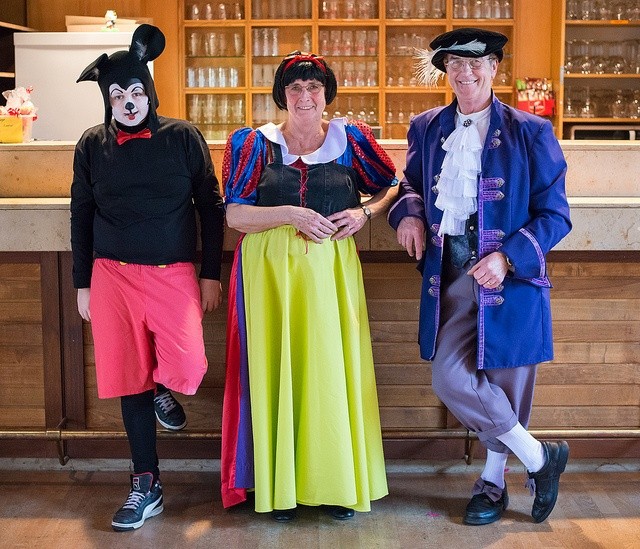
[111,472,165,532]
[151,390,187,430]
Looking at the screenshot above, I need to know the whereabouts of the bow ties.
[117,128,152,144]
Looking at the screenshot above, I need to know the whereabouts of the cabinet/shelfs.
[177,0,640,151]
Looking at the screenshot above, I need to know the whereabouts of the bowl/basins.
[0,115,37,144]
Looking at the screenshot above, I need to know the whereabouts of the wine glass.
[324,96,377,122]
[387,61,441,123]
[565,0,640,118]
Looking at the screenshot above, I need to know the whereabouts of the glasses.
[285,82,325,95]
[445,57,495,71]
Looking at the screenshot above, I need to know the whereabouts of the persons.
[70,22,226,533]
[386,27,573,526]
[220,51,403,522]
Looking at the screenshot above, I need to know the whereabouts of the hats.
[412,27,507,89]
[76,24,164,147]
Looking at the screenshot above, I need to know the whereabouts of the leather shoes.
[331,503,354,519]
[464,477,510,525]
[525,438,570,522]
[271,509,295,522]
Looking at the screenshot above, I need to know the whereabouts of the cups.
[189,95,287,142]
[387,0,512,19]
[188,0,378,88]
[386,33,442,56]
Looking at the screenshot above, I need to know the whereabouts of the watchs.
[360,204,371,220]
[504,253,512,269]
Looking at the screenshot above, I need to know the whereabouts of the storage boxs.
[14,32,154,141]
